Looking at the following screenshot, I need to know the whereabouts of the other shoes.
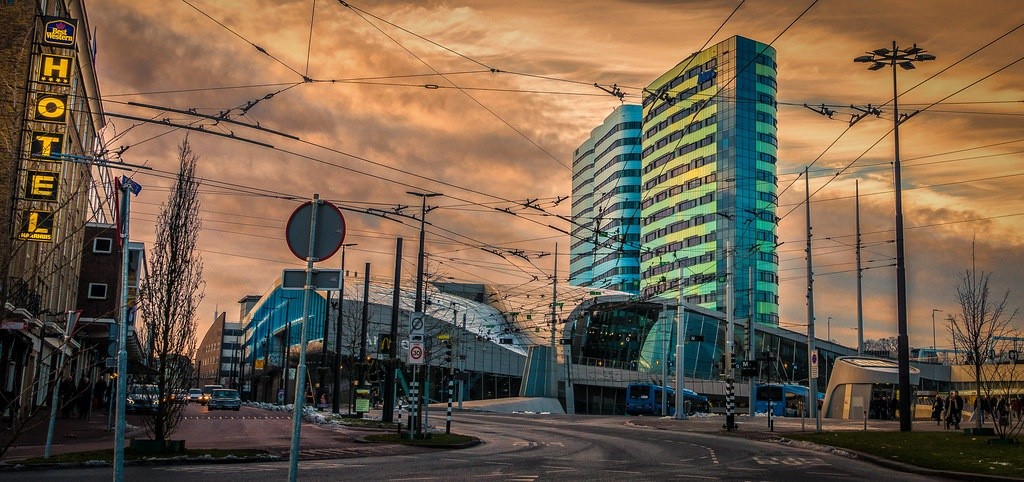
[955,425,960,429]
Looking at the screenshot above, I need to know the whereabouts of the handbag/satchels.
[951,417,957,425]
[934,400,938,408]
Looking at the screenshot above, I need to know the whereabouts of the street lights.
[851,35,937,435]
[827,317,836,342]
[332,243,359,412]
[405,190,444,439]
[932,309,944,349]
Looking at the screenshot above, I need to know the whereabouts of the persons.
[321,393,326,412]
[60,376,117,420]
[878,395,899,421]
[708,400,712,412]
[945,391,963,429]
[933,395,943,425]
[306,388,315,407]
[974,393,1024,423]
[791,402,797,416]
[372,391,376,408]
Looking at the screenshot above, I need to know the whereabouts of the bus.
[753,383,826,417]
[624,381,712,415]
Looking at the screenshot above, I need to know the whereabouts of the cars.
[187,389,202,403]
[127,384,159,414]
[207,388,242,411]
[170,388,188,404]
[200,385,224,406]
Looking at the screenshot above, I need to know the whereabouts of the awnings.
[98,358,160,375]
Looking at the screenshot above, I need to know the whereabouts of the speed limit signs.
[410,347,423,360]
